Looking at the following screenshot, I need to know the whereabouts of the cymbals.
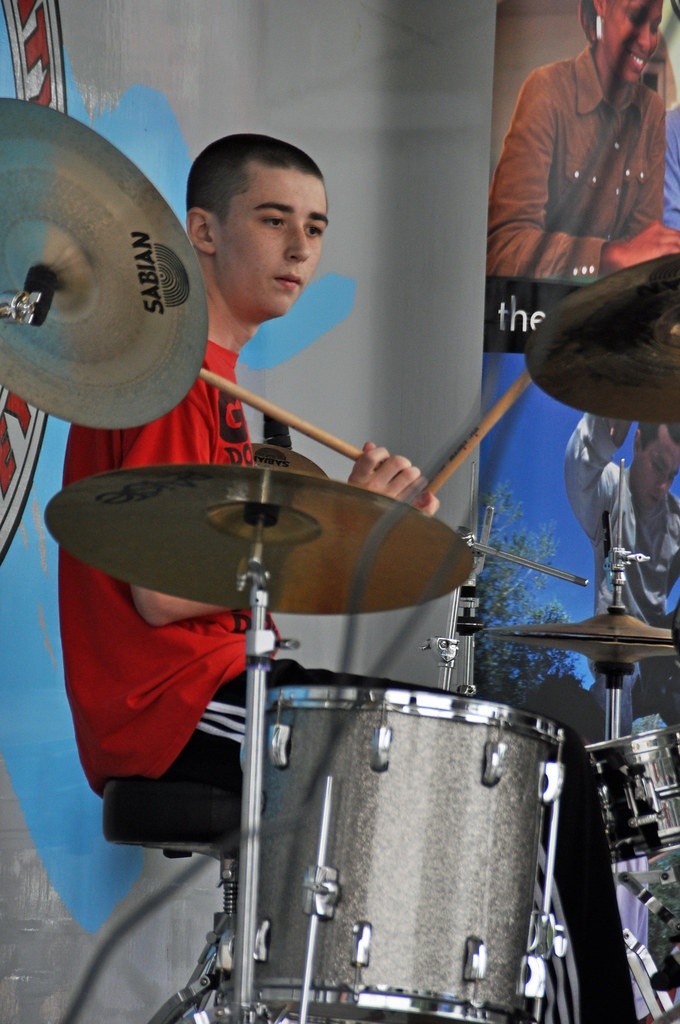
[42,461,476,617]
[524,251,680,425]
[482,614,678,662]
[0,97,209,431]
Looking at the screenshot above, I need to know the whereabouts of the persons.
[486,0,680,283]
[518,669,648,1024]
[564,405,680,738]
[58,133,639,1024]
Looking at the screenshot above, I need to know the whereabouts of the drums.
[584,726,680,864]
[252,686,566,1024]
